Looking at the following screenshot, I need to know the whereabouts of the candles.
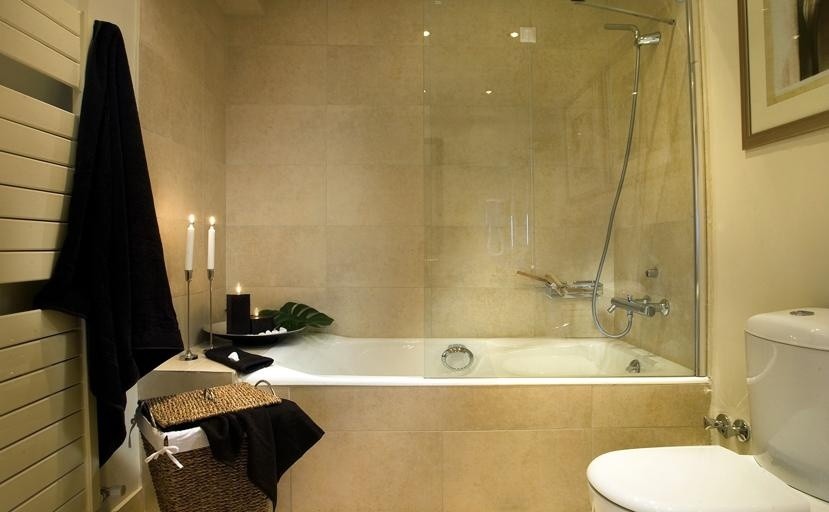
[184,213,195,271]
[226,282,250,333]
[208,216,215,270]
[250,307,274,334]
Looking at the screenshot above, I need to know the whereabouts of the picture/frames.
[738,0,829,150]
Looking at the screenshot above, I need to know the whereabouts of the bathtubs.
[236,330,713,385]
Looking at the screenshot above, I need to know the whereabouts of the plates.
[200,320,307,348]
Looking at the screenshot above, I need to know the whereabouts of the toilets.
[586,307,828,512]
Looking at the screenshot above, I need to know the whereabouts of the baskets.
[136,379,281,512]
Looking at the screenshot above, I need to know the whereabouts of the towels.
[204,345,273,374]
[40,19,184,470]
[181,399,324,512]
